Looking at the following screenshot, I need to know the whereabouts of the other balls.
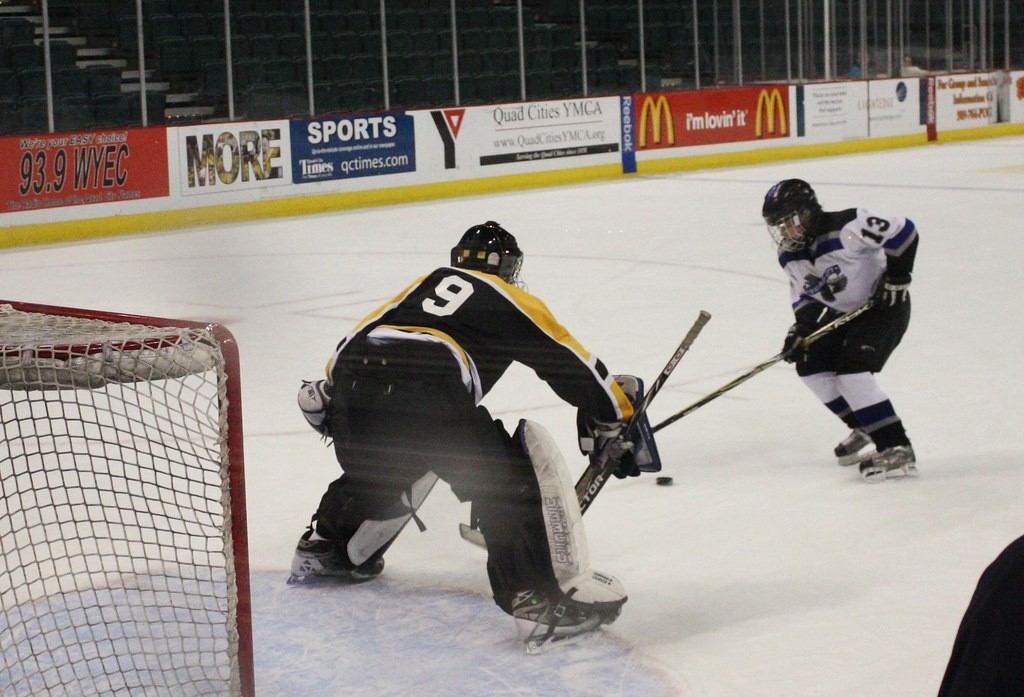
[656,477,673,486]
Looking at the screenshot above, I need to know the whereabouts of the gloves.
[298,379,334,440]
[782,321,819,364]
[869,272,913,315]
[594,418,641,479]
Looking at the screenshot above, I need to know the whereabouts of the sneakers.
[833,424,880,466]
[287,530,385,585]
[859,414,919,483]
[511,588,604,654]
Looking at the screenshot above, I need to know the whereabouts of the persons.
[762,179,920,483]
[845,51,875,77]
[285,221,643,655]
[904,55,925,73]
[938,534,1024,697]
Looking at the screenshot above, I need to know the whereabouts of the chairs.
[0,0,1024,134]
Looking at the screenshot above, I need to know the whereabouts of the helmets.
[762,178,820,252]
[451,220,522,283]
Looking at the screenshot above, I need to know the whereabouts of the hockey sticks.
[458,307,707,599]
[649,300,874,434]
